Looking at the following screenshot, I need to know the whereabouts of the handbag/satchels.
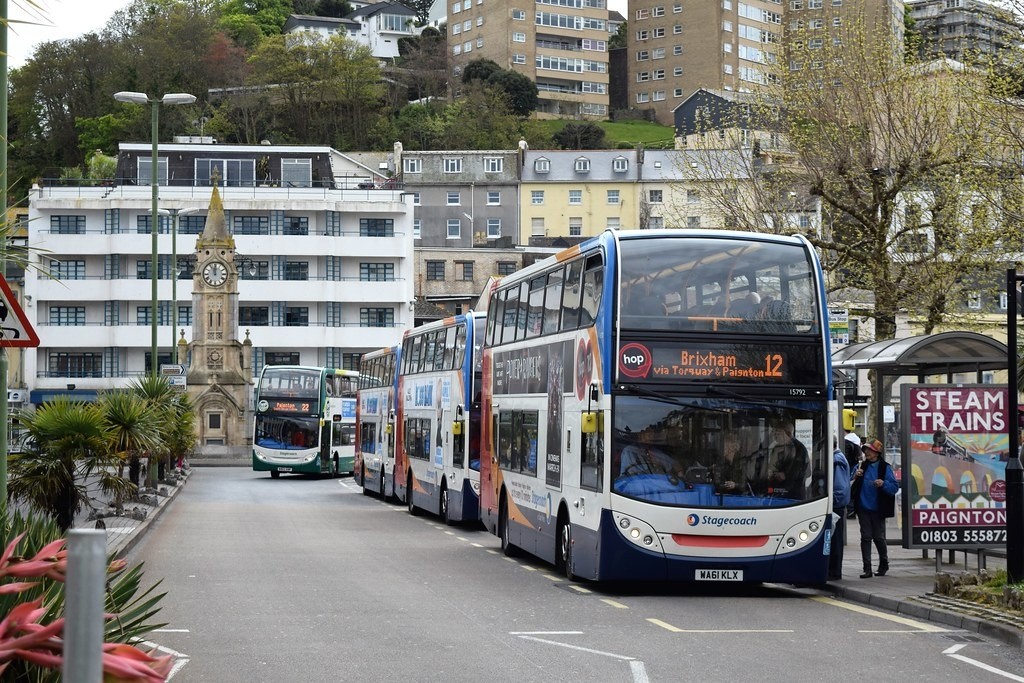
[877,493,895,517]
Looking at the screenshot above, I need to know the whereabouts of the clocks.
[203,262,228,287]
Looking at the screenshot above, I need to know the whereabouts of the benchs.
[923,548,1007,571]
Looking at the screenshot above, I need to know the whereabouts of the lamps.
[176,254,198,276]
[233,255,257,276]
[67,384,75,390]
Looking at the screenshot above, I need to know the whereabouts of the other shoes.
[860,571,872,577]
[874,564,889,576]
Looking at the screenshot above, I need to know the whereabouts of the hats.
[861,438,884,454]
[833,434,838,442]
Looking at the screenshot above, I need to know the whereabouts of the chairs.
[620,297,796,333]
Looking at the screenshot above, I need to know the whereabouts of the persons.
[518,136,530,150]
[849,438,900,578]
[844,429,867,519]
[286,381,332,447]
[826,435,851,581]
[507,283,815,501]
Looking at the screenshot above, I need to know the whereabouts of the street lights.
[147,206,200,368]
[113,89,197,384]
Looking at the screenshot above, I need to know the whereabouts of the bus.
[354,347,403,502]
[398,309,482,526]
[252,364,382,479]
[482,227,840,598]
